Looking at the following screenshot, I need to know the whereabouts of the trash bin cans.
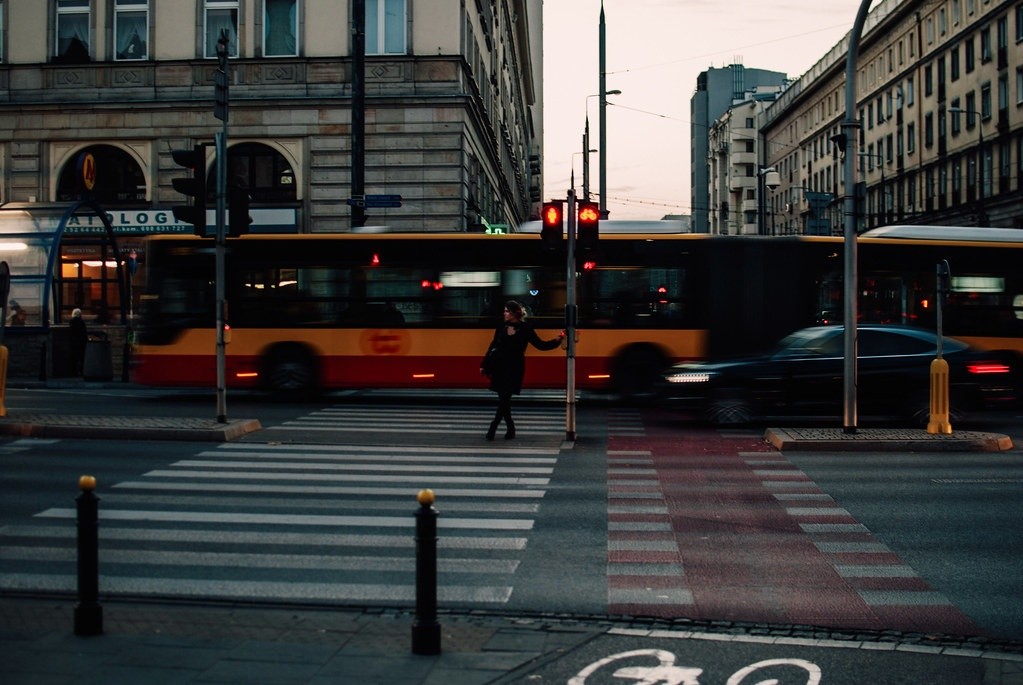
[82,330,115,383]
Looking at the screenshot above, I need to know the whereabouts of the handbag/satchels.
[484,347,496,377]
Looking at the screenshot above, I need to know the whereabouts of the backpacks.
[16,308,27,321]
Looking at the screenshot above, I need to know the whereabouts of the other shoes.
[505,430,516,440]
[486,432,495,440]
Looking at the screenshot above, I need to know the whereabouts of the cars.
[654,322,1023,432]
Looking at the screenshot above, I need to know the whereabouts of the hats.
[72,308,82,319]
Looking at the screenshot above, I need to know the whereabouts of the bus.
[122,220,1023,445]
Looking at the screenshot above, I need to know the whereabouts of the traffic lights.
[170,144,207,239]
[576,201,599,272]
[540,202,564,268]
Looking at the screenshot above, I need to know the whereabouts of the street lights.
[581,89,623,204]
[567,149,598,200]
[946,107,985,228]
[857,151,886,227]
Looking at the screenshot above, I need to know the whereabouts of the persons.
[5,299,20,320]
[68,308,88,379]
[480,301,566,441]
[10,310,27,326]
